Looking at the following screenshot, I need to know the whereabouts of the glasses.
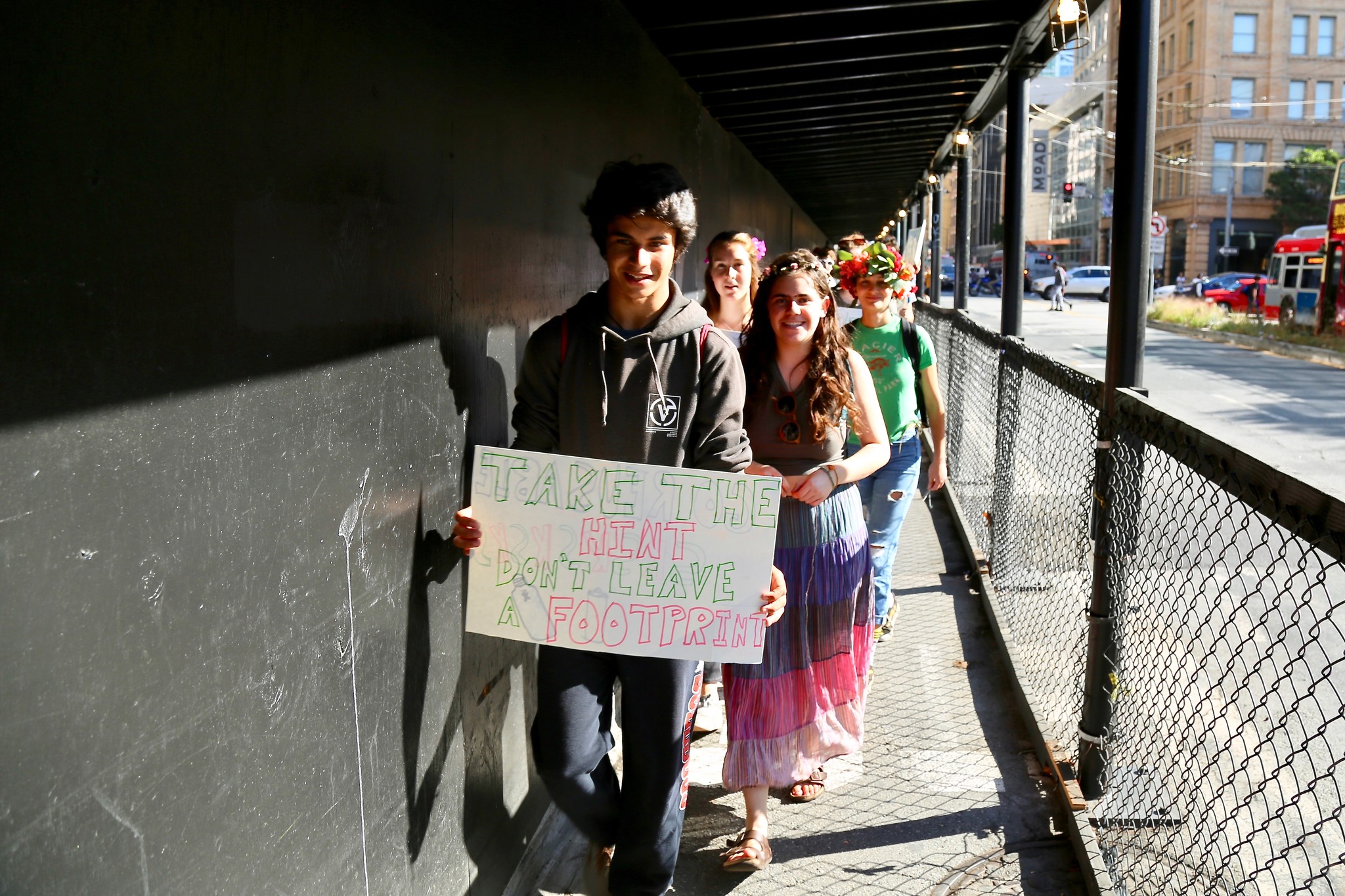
[774,389,801,445]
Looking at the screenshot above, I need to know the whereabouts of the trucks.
[925,254,956,289]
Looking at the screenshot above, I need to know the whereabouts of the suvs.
[1031,265,1111,303]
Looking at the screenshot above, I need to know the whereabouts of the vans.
[969,266,987,284]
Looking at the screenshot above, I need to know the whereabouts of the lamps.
[925,168,944,195]
[1041,0,1094,55]
[948,125,975,160]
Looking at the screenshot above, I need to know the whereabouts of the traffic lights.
[1063,182,1073,202]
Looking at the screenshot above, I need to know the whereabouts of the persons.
[455,145,788,896]
[969,268,1001,294]
[717,248,892,873]
[1049,260,1073,312]
[1247,276,1262,323]
[691,230,767,736]
[837,242,950,646]
[1176,271,1185,285]
[1191,272,1207,300]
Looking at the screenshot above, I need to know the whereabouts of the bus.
[1261,224,1328,332]
[1311,158,1344,338]
[989,250,1059,281]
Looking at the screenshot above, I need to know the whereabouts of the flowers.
[701,244,711,266]
[828,243,920,299]
[751,233,769,262]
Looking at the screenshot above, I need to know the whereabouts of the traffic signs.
[1218,246,1240,257]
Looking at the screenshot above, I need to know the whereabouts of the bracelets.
[826,465,838,488]
[818,467,836,492]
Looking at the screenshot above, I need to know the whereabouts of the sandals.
[718,829,772,872]
[789,764,828,801]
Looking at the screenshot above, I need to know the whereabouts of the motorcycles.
[969,271,1002,298]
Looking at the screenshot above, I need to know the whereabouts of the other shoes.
[586,837,615,880]
[878,592,900,642]
[867,665,874,695]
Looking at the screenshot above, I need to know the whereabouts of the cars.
[1153,272,1279,322]
